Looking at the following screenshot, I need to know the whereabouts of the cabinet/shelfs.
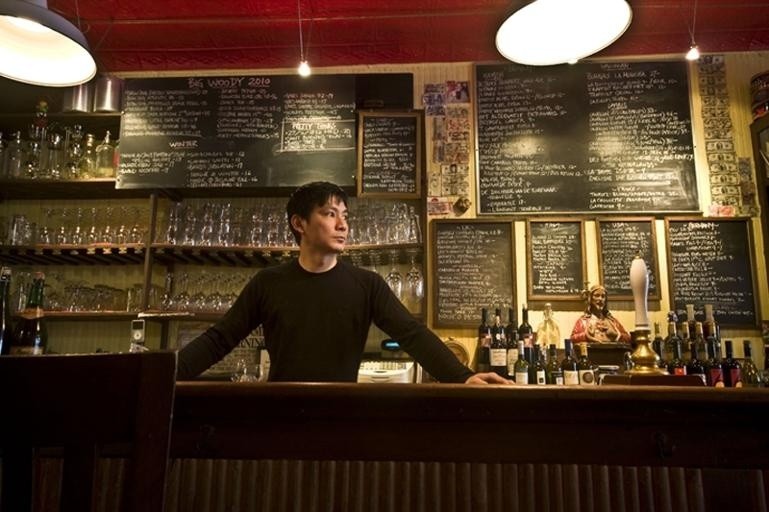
[1,110,120,183]
[1,186,427,387]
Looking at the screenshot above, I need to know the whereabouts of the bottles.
[652,302,769,388]
[476,301,597,387]
[1,107,119,183]
[0,265,48,356]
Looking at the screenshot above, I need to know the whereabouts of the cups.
[61,76,123,115]
[228,358,264,384]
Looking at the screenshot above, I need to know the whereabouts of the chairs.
[1,348,181,512]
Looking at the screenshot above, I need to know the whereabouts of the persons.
[447,81,455,96]
[537,301,562,350]
[570,283,632,344]
[461,82,468,97]
[447,83,468,101]
[177,180,520,388]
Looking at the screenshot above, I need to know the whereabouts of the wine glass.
[149,248,426,314]
[1,270,144,314]
[158,196,424,244]
[1,197,152,247]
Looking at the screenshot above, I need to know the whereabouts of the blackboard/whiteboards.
[528,219,588,301]
[473,58,703,216]
[665,218,761,330]
[114,74,356,190]
[596,219,662,301]
[357,111,422,200]
[432,219,517,331]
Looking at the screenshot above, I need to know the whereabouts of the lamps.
[0,0,97,88]
[495,0,633,67]
